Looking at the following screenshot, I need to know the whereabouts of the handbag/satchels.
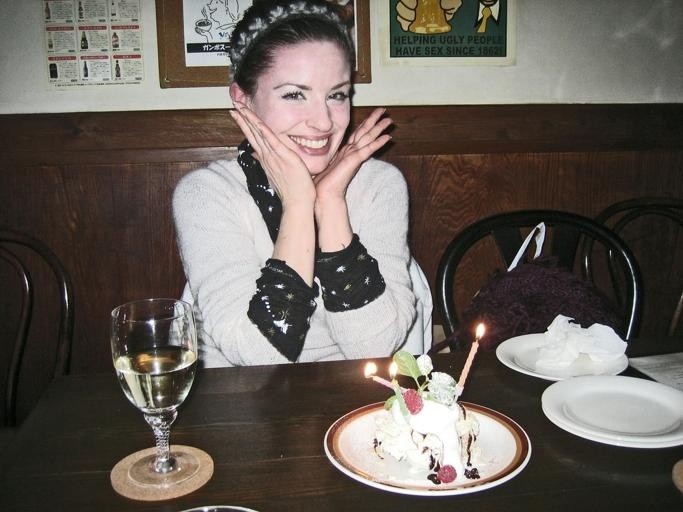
[427,221,626,358]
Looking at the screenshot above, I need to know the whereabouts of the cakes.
[369,349,481,486]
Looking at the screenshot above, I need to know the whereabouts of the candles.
[364,360,410,420]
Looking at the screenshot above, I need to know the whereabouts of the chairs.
[1,225,77,449]
[435,195,682,354]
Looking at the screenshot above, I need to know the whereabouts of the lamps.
[454,322,488,399]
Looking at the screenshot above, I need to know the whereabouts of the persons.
[165,1,439,369]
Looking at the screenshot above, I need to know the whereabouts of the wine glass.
[109,297,201,490]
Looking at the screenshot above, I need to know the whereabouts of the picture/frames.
[155,1,373,89]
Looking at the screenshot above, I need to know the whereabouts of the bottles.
[49,63,58,78]
[115,59,121,78]
[78,1,84,19]
[111,1,116,20]
[44,2,51,20]
[80,31,88,49]
[83,61,89,79]
[47,32,55,54]
[112,32,120,50]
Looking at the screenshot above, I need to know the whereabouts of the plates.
[495,331,629,381]
[541,374,682,449]
[323,398,533,498]
